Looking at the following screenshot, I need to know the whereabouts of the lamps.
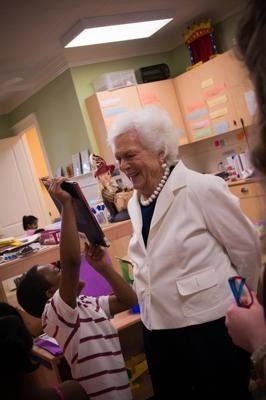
[60,9,174,49]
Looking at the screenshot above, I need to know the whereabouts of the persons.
[226,6,266,383]
[108,105,262,400]
[16,175,138,400]
[22,215,38,236]
[0,302,64,400]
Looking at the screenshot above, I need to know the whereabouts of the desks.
[31,302,141,394]
[0,220,136,304]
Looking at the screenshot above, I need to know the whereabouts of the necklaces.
[140,163,170,206]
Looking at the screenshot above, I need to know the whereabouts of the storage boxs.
[91,69,136,91]
[228,181,266,225]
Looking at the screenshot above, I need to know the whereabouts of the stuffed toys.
[90,154,135,216]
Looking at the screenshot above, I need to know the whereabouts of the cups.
[95,211,111,225]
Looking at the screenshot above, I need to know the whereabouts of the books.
[40,177,110,248]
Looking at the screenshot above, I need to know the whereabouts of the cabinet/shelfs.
[173,47,262,144]
[85,78,190,167]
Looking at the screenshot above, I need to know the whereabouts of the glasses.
[228,276,253,308]
